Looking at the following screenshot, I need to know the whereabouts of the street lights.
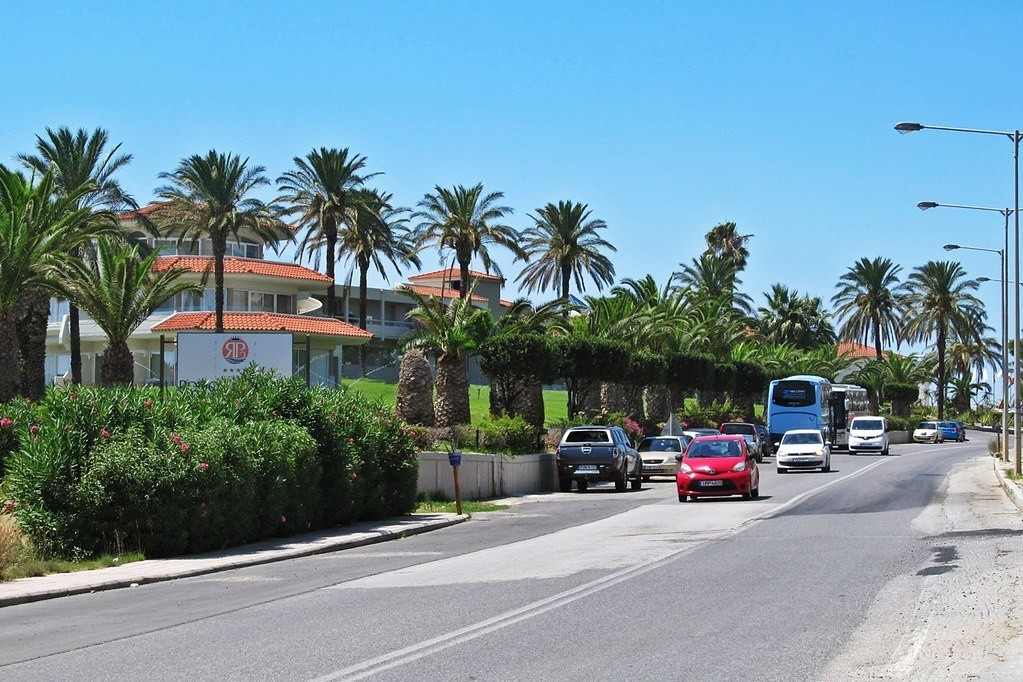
[893,122,1022,477]
[915,202,1014,464]
[941,244,1004,458]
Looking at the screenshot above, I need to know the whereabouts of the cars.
[755,425,772,457]
[913,421,944,444]
[939,421,966,443]
[682,431,703,441]
[687,428,720,436]
[637,435,690,483]
[774,430,832,474]
[675,435,760,502]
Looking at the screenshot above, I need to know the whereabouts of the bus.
[831,383,869,449]
[767,375,836,455]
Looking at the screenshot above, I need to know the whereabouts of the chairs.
[700,444,712,457]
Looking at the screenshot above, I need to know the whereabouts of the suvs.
[555,425,643,492]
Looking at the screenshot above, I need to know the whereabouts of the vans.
[846,416,891,456]
[719,422,765,463]
